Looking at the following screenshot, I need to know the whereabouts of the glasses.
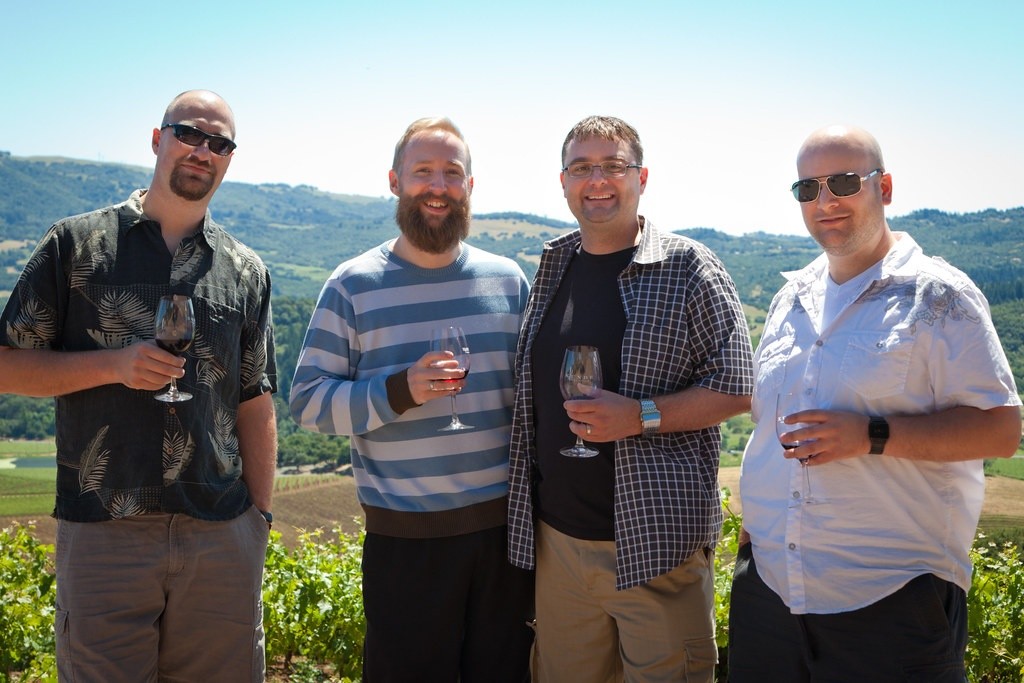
[790,167,881,201]
[562,159,643,176]
[160,123,236,155]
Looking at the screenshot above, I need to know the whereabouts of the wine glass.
[428,325,475,431]
[559,345,603,458]
[154,296,195,402]
[776,392,830,509]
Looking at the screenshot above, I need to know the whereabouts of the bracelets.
[258,507,273,530]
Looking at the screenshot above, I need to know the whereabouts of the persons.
[287,115,536,683]
[515,117,754,683]
[726,122,1022,683]
[0,89,276,683]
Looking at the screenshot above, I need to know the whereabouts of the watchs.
[640,393,662,437]
[863,416,890,456]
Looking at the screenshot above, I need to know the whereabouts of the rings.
[586,425,592,436]
[429,382,434,391]
